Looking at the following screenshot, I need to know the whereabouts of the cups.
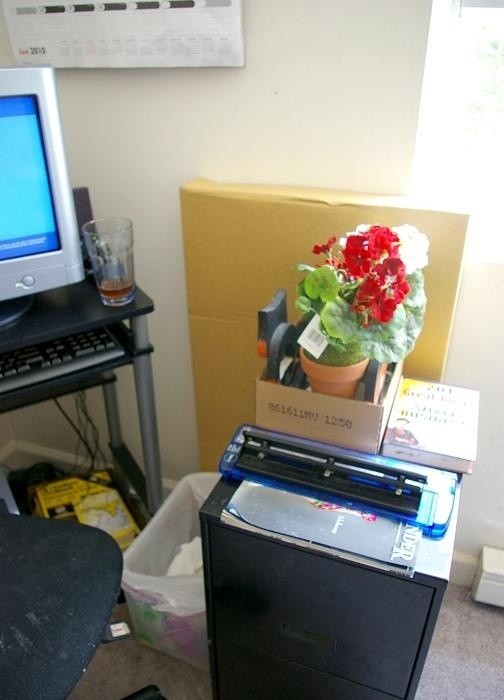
[81,218,135,307]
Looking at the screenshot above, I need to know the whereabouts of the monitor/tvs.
[0,66,87,305]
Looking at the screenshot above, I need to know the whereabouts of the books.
[219,480,424,579]
[382,377,480,475]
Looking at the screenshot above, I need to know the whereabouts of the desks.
[0,275,164,518]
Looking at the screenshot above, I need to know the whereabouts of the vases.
[292,222,430,367]
[299,345,369,398]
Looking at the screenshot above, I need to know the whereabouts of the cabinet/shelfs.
[198,471,462,700]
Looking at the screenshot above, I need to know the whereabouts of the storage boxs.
[180,175,471,472]
[256,354,403,455]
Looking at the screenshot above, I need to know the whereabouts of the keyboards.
[0,326,126,395]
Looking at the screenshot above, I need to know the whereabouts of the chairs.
[0,513,165,700]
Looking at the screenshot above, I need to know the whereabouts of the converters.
[7,470,38,516]
